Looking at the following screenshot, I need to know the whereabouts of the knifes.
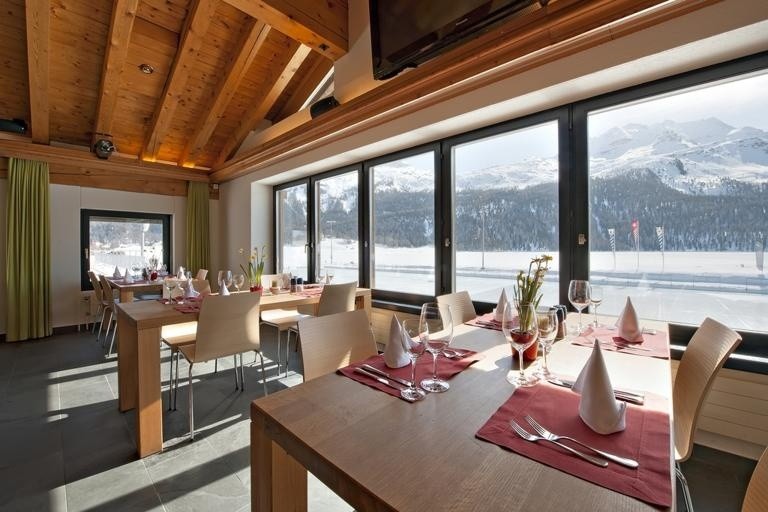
[548,377,645,407]
[355,363,413,391]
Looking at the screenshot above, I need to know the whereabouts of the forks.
[509,413,641,470]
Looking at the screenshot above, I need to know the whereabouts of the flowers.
[510,254,554,335]
[238,243,271,288]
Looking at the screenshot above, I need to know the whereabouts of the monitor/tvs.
[368,0,545,80]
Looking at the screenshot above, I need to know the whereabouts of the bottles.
[291,277,304,293]
[551,304,567,339]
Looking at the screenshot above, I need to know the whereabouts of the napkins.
[176,268,187,281]
[113,266,122,279]
[383,312,413,368]
[185,276,201,297]
[616,295,644,343]
[219,280,230,296]
[123,268,136,283]
[493,285,514,325]
[570,336,632,434]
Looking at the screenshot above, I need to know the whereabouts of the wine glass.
[499,303,559,388]
[217,270,244,293]
[568,278,606,342]
[161,277,178,306]
[396,301,454,403]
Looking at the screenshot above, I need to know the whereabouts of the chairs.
[674,315,744,511]
[87,268,109,357]
[284,280,359,380]
[98,273,143,357]
[258,273,314,378]
[292,307,381,382]
[742,447,768,512]
[433,290,476,334]
[161,279,212,411]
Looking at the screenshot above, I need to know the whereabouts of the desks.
[248,302,680,512]
[117,282,372,459]
[105,277,181,322]
